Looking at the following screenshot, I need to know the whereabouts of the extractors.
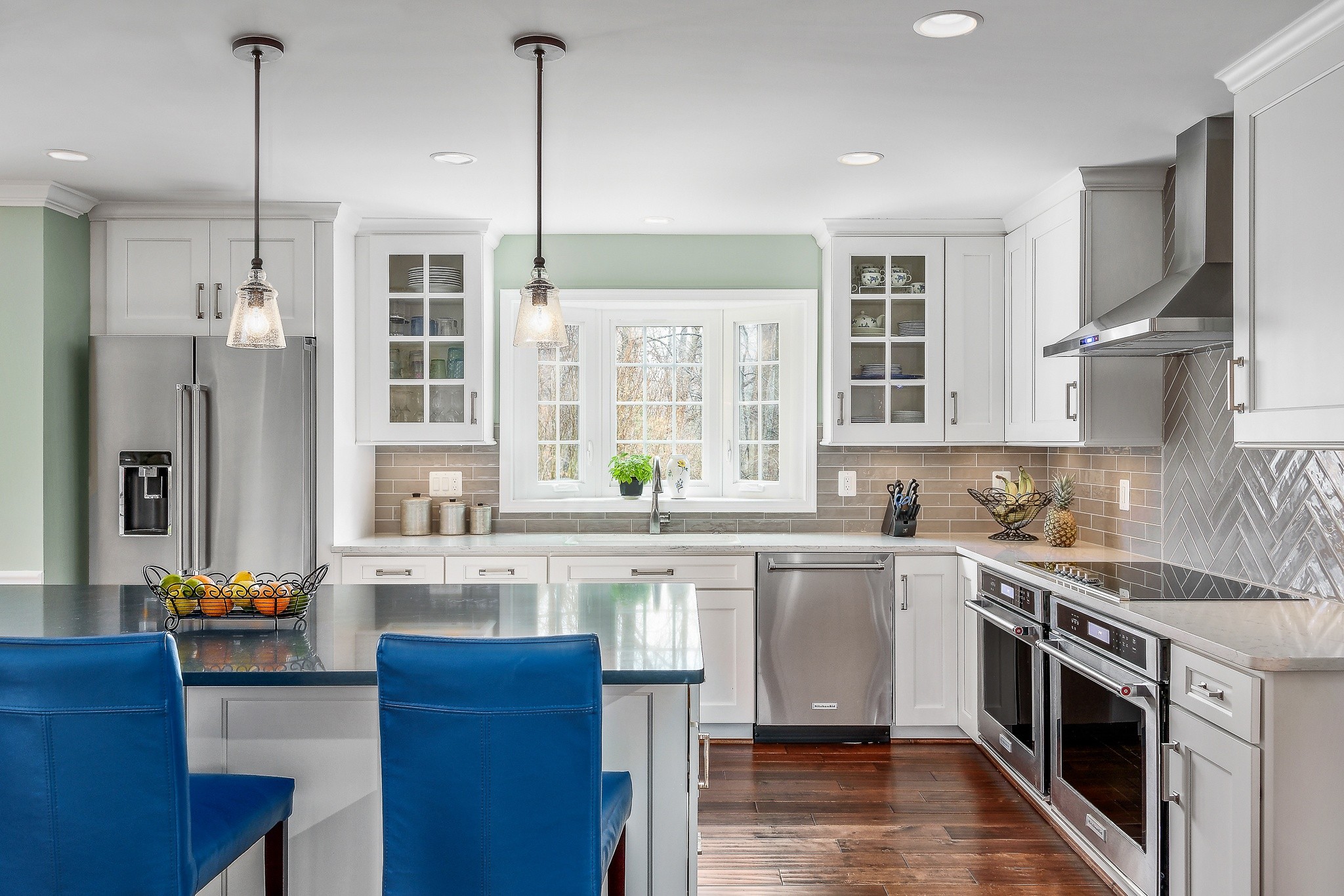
[1042,115,1234,357]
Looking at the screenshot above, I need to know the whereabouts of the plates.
[860,363,902,376]
[851,417,885,423]
[891,410,924,423]
[897,320,925,336]
[851,328,885,336]
[408,266,462,293]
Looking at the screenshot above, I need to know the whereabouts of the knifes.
[887,478,921,520]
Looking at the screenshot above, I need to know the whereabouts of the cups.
[469,503,492,534]
[390,348,423,379]
[908,283,925,294]
[400,493,433,535]
[439,499,467,535]
[429,347,464,379]
[857,263,912,286]
[390,313,464,336]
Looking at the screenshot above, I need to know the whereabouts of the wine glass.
[429,390,464,422]
[391,390,424,422]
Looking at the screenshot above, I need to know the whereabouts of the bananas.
[991,475,1018,518]
[1006,465,1035,523]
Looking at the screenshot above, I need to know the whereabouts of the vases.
[665,454,690,499]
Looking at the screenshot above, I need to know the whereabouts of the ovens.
[964,562,1051,809]
[1035,594,1170,896]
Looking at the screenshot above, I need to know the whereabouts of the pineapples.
[1044,469,1077,548]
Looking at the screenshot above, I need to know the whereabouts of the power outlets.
[428,472,462,497]
[993,471,1011,496]
[838,470,857,497]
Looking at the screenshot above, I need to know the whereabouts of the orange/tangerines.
[200,584,234,616]
[254,582,289,615]
[190,575,216,589]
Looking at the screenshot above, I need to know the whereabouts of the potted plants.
[607,451,655,496]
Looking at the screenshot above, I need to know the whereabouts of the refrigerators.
[87,334,315,587]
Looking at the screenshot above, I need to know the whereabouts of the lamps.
[513,35,570,349]
[225,35,287,349]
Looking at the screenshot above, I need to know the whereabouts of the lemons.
[158,572,309,615]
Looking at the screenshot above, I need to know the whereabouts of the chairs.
[0,626,296,896]
[376,630,632,896]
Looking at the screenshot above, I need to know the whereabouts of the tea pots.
[851,310,885,327]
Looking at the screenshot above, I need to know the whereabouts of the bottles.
[666,454,691,499]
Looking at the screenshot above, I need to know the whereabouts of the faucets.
[649,455,671,534]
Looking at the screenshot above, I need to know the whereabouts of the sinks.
[565,534,742,546]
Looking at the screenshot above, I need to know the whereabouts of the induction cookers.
[1016,560,1309,603]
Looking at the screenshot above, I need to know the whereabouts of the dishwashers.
[754,552,894,743]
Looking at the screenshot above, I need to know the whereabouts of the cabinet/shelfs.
[1213,24,1344,453]
[178,686,710,896]
[1161,640,1344,896]
[890,552,982,744]
[810,190,1166,447]
[354,233,504,447]
[105,219,316,337]
[340,554,756,739]
